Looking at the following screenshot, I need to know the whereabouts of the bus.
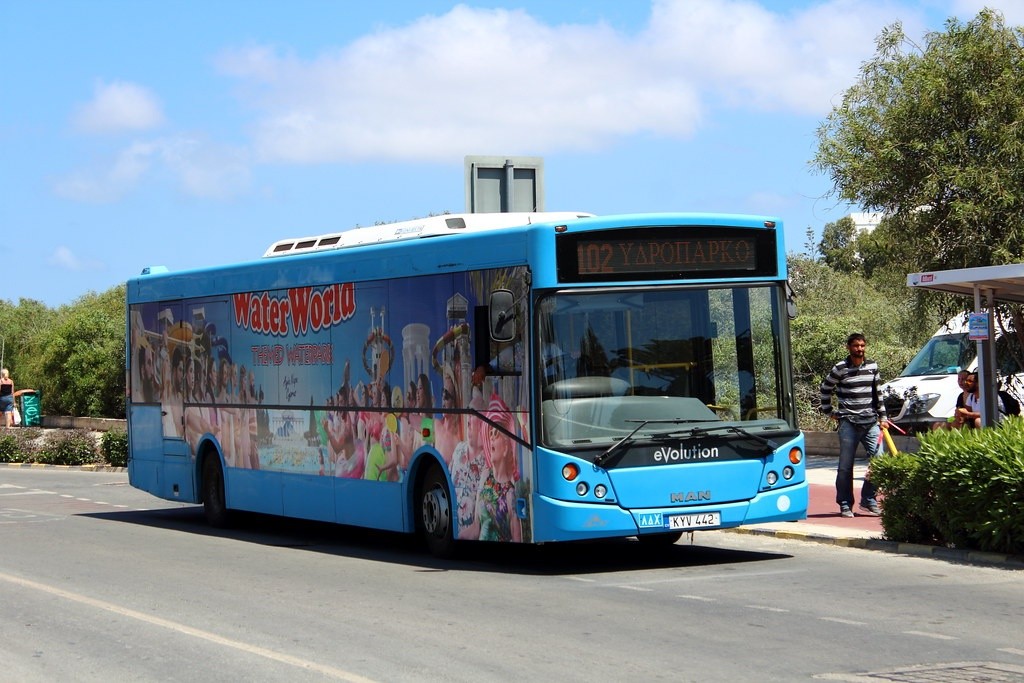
[126,212,809,559]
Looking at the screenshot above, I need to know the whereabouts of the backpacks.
[997,391,1020,417]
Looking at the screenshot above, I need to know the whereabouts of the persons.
[933,370,1006,431]
[0,369,15,428]
[470,325,579,385]
[129,313,258,469]
[317,358,522,543]
[820,332,887,518]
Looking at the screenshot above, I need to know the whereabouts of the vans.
[880,306,1024,436]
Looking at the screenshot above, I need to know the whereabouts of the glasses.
[443,387,453,400]
[489,427,501,439]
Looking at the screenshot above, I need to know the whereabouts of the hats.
[445,362,459,405]
[481,395,521,481]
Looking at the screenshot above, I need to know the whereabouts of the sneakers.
[859,495,882,514]
[839,499,855,518]
